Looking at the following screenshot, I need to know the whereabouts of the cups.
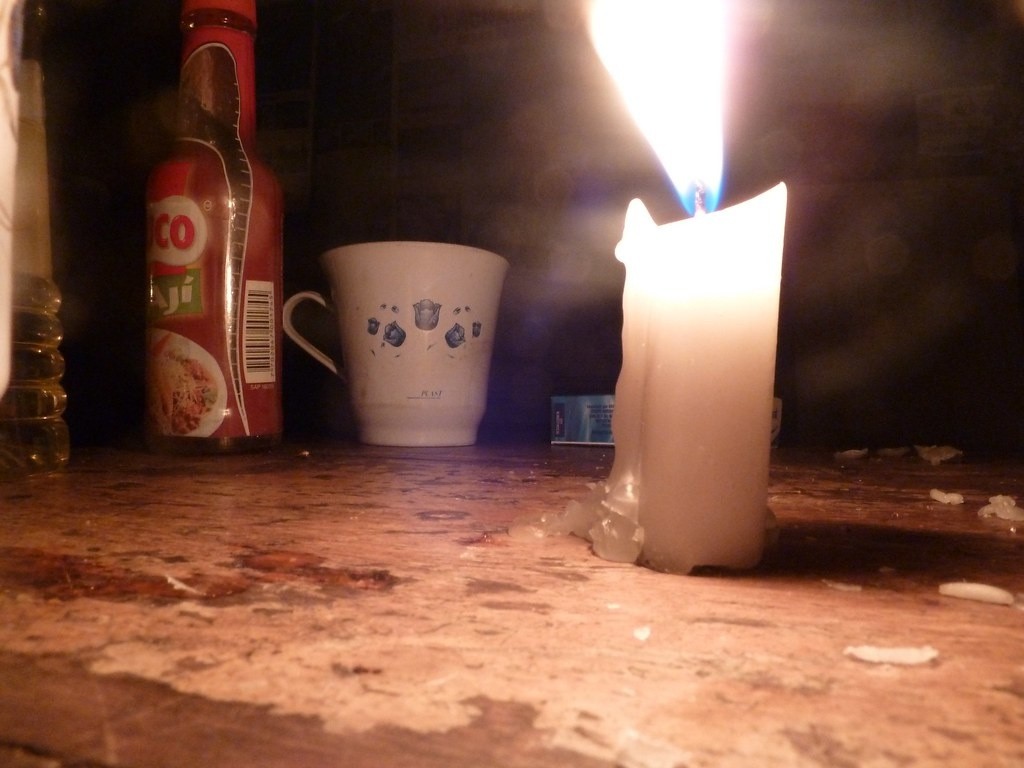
[281,239,511,447]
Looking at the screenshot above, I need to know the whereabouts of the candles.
[558,1,788,579]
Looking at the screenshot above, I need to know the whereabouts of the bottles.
[144,0,282,454]
[1,2,69,493]
